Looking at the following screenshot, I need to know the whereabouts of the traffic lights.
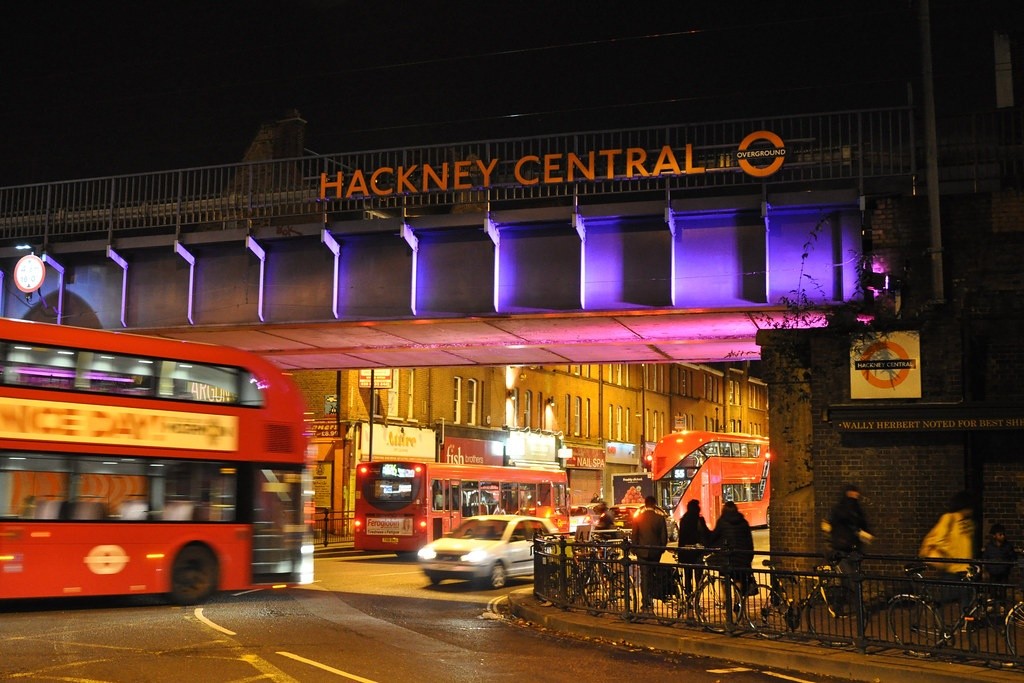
[646,455,653,473]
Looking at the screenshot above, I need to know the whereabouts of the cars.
[417,514,568,591]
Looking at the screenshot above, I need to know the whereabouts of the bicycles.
[646,543,746,634]
[886,562,1024,671]
[1005,600,1024,668]
[541,537,639,623]
[744,539,869,648]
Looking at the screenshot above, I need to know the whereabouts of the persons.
[591,492,600,503]
[918,505,976,632]
[677,500,708,594]
[821,487,879,622]
[630,495,666,608]
[710,501,754,599]
[594,513,626,538]
[979,522,1017,629]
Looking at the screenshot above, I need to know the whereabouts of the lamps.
[508,390,515,400]
[548,396,555,406]
[13,240,33,250]
[883,273,904,295]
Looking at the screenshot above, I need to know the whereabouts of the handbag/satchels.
[740,571,759,596]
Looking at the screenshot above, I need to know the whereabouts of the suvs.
[568,503,608,537]
[609,502,679,542]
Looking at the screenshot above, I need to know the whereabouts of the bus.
[649,431,770,543]
[0,316,318,605]
[353,460,571,561]
